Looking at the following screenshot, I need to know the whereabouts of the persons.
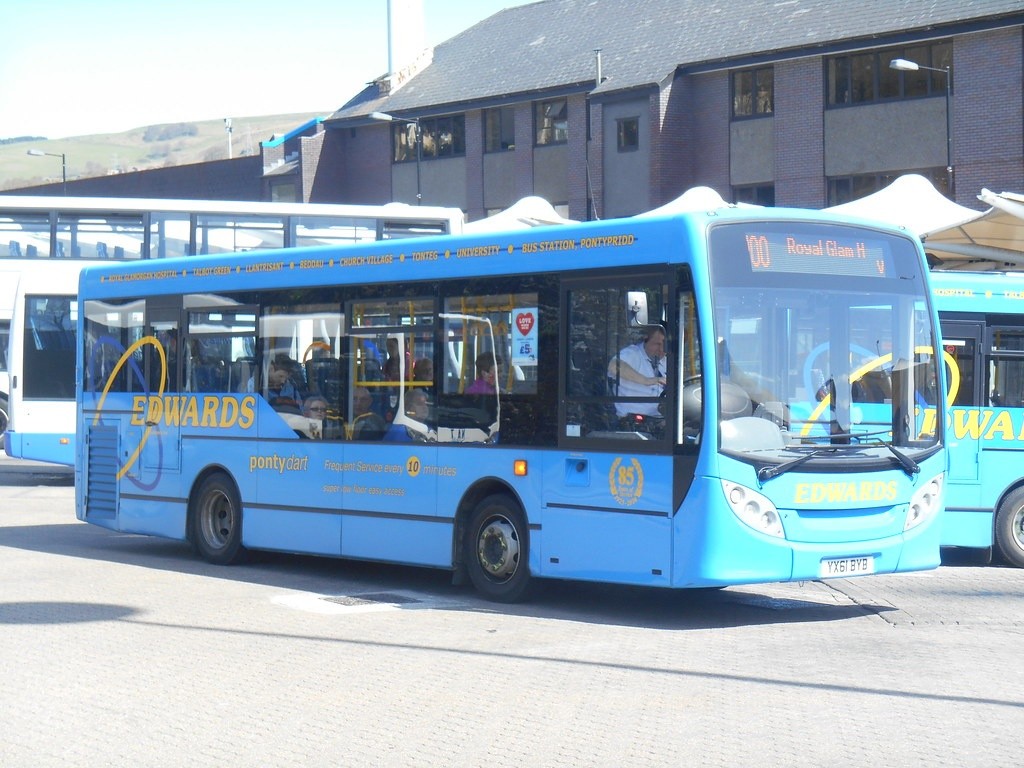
[605,320,672,437]
[463,350,514,401]
[82,310,443,446]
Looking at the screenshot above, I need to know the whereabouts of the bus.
[1,195,466,464]
[791,265,1024,566]
[69,198,948,611]
[10,271,74,469]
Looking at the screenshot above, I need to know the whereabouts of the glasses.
[310,408,328,412]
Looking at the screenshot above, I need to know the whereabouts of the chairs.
[114,246,124,258]
[30,310,76,351]
[9,241,22,257]
[56,241,65,258]
[103,243,108,258]
[234,354,383,406]
[76,247,81,257]
[96,241,106,258]
[349,412,387,440]
[185,244,189,252]
[140,243,144,259]
[25,244,38,256]
[200,248,208,254]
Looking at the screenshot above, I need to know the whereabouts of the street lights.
[890,57,955,201]
[27,149,68,195]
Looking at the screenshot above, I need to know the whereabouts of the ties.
[650,361,663,378]
